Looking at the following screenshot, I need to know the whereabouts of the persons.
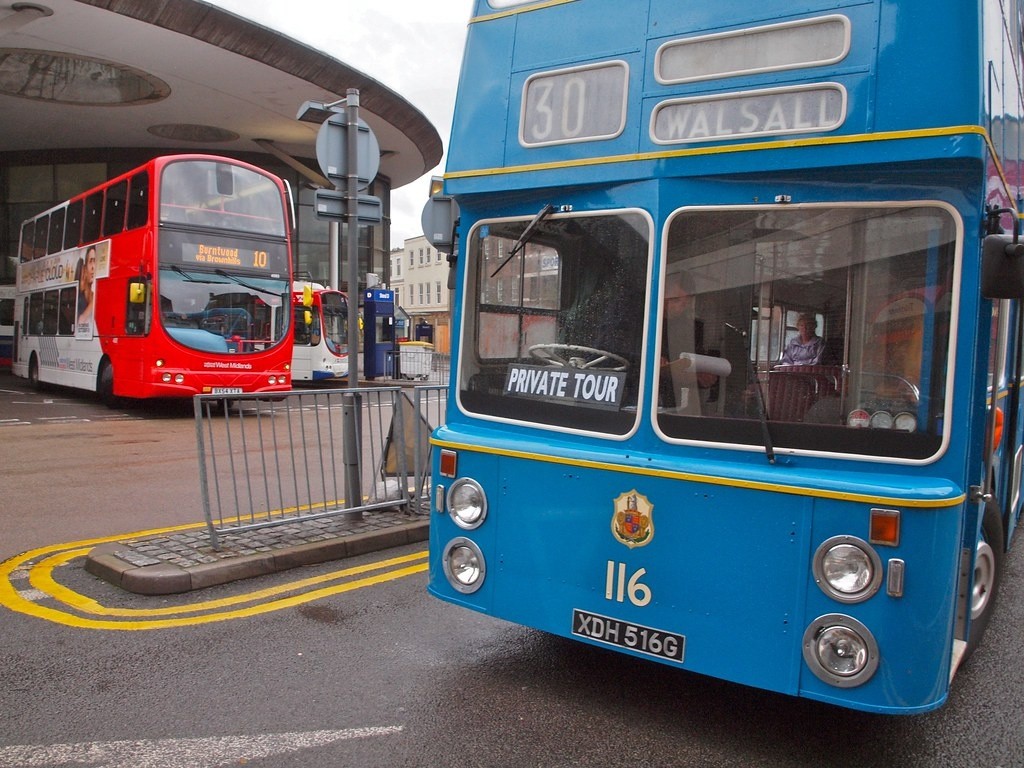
[659,271,714,405]
[781,315,832,365]
[74,246,98,325]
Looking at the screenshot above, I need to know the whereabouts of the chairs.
[747,364,920,423]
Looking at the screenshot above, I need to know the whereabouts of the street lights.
[295,86,366,523]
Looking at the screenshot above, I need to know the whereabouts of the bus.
[160,307,254,353]
[0,284,15,369]
[11,154,295,411]
[419,0,1024,718]
[290,278,348,381]
